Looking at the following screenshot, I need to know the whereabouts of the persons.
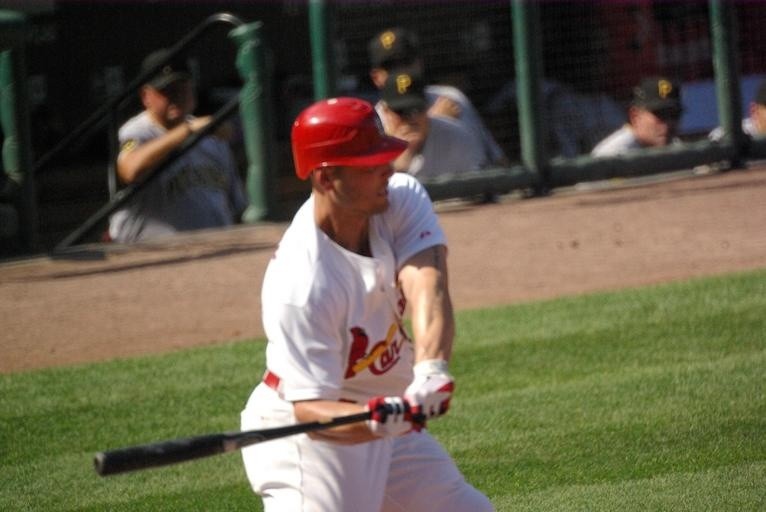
[241,96,497,511]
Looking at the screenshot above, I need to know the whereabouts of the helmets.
[290,96,409,180]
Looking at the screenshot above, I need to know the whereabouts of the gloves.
[364,359,456,437]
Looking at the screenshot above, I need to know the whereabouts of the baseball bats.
[92,401,412,475]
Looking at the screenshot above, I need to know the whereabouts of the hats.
[141,48,186,90]
[633,76,681,113]
[368,27,428,108]
